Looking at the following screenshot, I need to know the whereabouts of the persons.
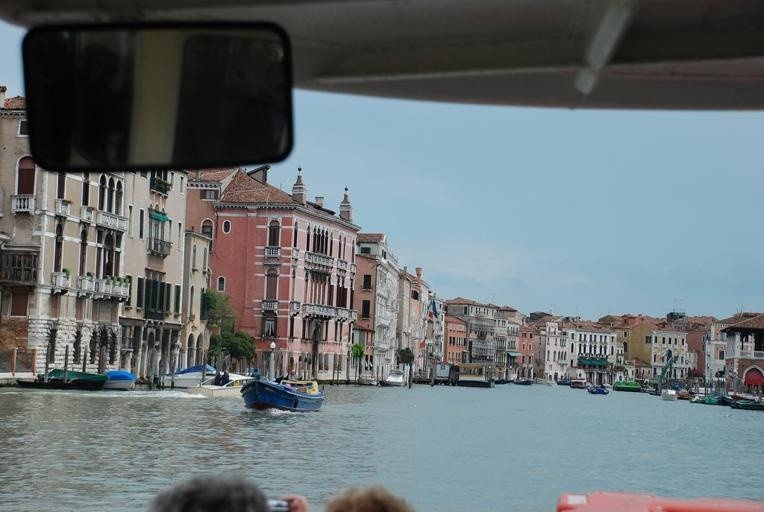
[135,375,159,384]
[148,475,308,512]
[215,370,229,386]
[325,484,415,512]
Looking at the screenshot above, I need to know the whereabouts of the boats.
[557,375,612,395]
[358,370,407,386]
[240,380,326,412]
[15,363,279,396]
[613,372,764,410]
[513,377,535,385]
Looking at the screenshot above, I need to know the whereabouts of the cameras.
[268,499,288,511]
